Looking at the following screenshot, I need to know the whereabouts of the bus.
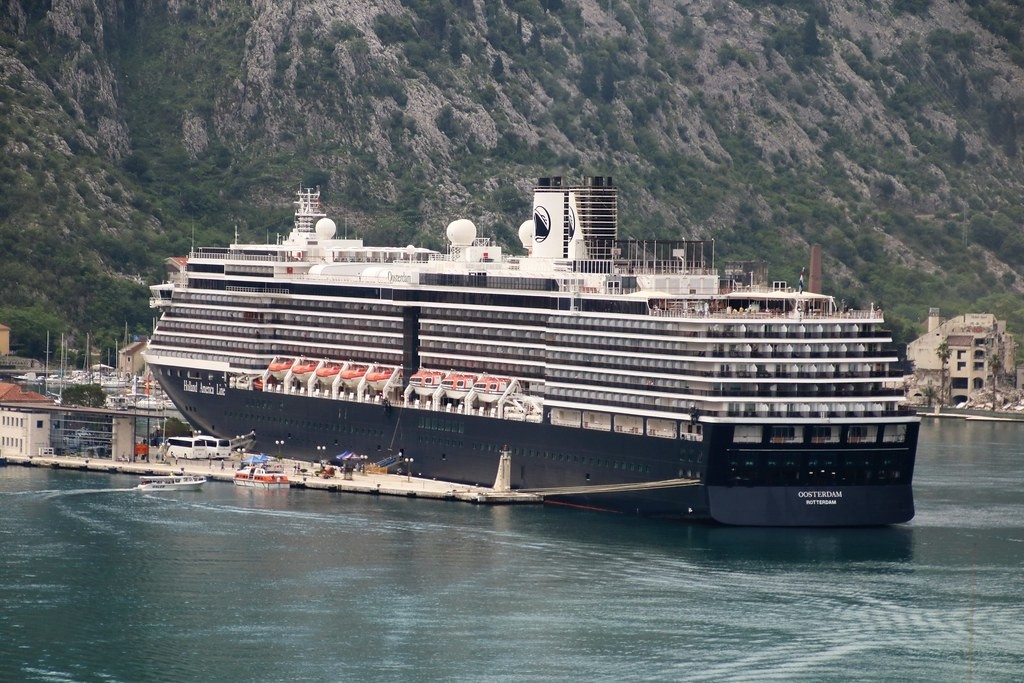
[195,435,231,460]
[162,436,207,460]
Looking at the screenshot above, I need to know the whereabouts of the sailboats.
[43,318,177,411]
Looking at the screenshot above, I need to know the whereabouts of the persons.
[208,453,212,469]
[232,460,234,468]
[176,455,178,464]
[221,460,224,469]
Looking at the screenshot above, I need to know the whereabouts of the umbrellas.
[241,454,277,466]
[336,450,361,471]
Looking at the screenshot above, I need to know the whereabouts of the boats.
[269,356,293,381]
[235,379,249,388]
[30,376,45,382]
[293,359,319,382]
[137,474,205,491]
[316,363,342,385]
[440,371,476,400]
[339,365,368,387]
[365,369,403,392]
[13,375,28,380]
[232,462,291,490]
[408,369,444,397]
[253,379,274,389]
[473,376,511,403]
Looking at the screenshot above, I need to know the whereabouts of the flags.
[799,267,805,294]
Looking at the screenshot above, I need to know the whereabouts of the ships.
[140,171,922,530]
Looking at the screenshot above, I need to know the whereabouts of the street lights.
[405,458,414,483]
[360,455,368,476]
[194,430,201,436]
[237,447,246,464]
[275,440,284,464]
[236,435,244,450]
[317,446,327,470]
